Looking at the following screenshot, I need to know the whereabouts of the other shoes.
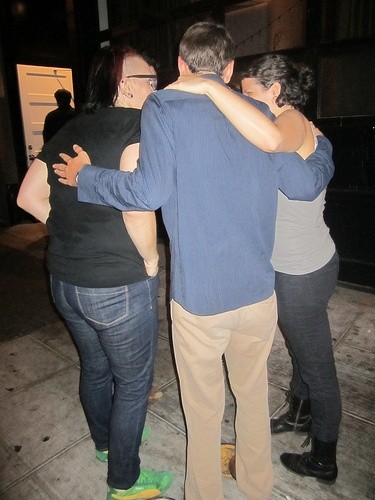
[229,455,237,479]
[149,386,164,399]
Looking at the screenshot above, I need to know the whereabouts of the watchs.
[75,171,79,184]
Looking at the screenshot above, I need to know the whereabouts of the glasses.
[126,75,158,91]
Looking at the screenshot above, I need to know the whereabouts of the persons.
[52,20,334,500]
[14,43,175,499]
[164,54,343,485]
[42,89,80,145]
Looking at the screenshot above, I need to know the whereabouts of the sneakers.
[95,424,151,463]
[107,469,175,499]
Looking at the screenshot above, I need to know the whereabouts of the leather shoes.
[280,453,338,485]
[270,415,311,434]
